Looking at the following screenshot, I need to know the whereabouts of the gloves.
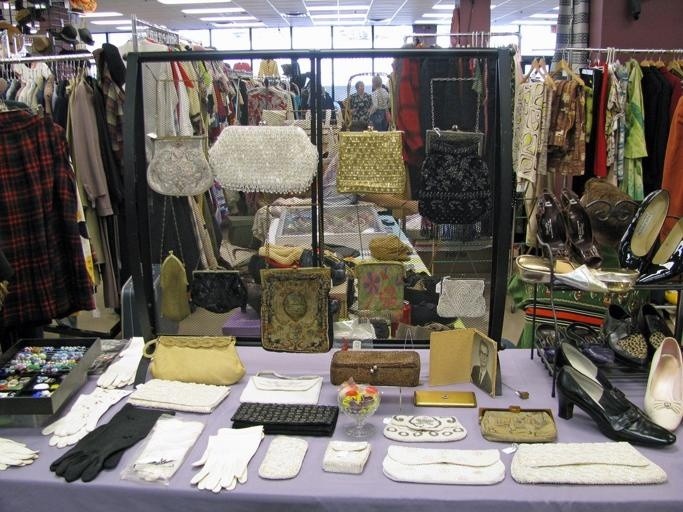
[193,422,264,497]
[42,387,120,448]
[51,405,154,482]
[98,334,146,390]
[1,434,41,474]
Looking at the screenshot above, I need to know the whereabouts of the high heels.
[551,343,627,405]
[635,236,682,288]
[555,365,677,448]
[617,188,669,268]
[643,337,683,430]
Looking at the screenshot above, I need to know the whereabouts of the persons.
[343,82,372,132]
[367,76,391,131]
[328,123,419,214]
[470,342,492,395]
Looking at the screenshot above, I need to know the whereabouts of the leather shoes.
[536,321,568,362]
[568,320,614,367]
[636,301,674,347]
[603,305,650,363]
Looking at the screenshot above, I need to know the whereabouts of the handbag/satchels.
[414,390,477,409]
[232,399,339,437]
[479,404,558,442]
[189,218,243,313]
[257,210,333,357]
[417,76,490,227]
[146,75,214,197]
[209,77,321,196]
[333,71,408,196]
[127,379,227,413]
[141,335,246,387]
[238,370,324,406]
[382,414,469,444]
[509,442,671,489]
[154,197,192,321]
[382,444,505,488]
[320,442,371,476]
[353,196,405,315]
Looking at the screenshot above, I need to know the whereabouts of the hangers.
[533,47,683,92]
[0,48,119,151]
[137,21,178,48]
[405,30,522,48]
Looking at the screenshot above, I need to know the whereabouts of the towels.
[131,413,203,486]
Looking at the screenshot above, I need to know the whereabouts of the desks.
[0,338,681,507]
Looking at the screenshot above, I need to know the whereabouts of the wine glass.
[337,382,382,439]
